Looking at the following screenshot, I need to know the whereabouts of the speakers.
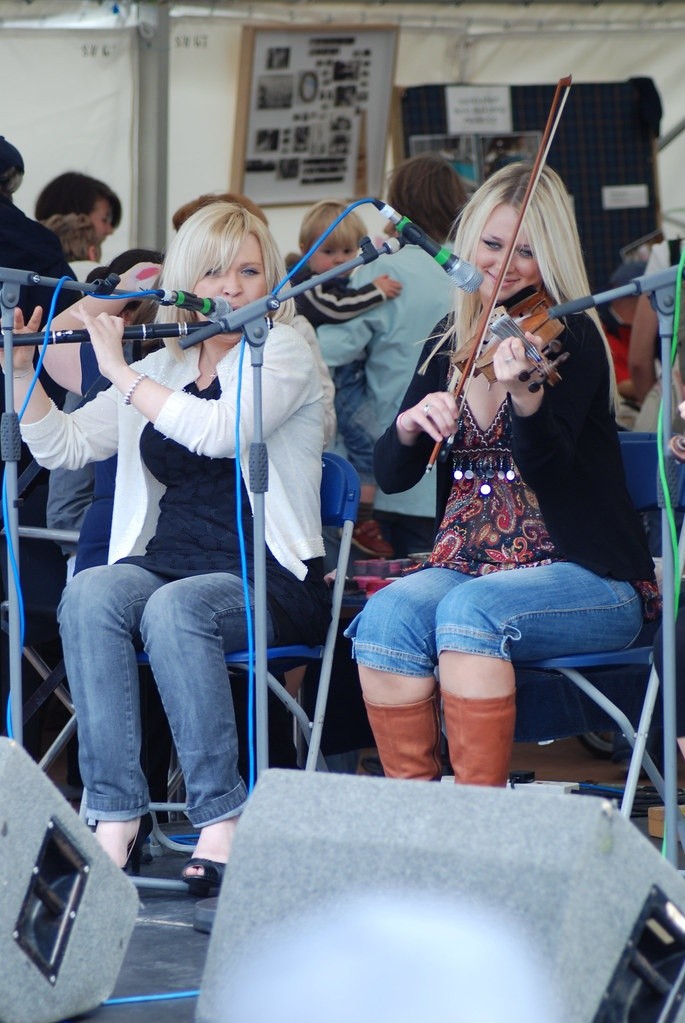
[196,767,685,1023]
[0,735,140,1023]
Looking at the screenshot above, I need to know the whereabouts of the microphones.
[373,198,484,292]
[155,288,235,323]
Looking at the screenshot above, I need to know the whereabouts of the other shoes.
[362,683,440,783]
[444,688,517,789]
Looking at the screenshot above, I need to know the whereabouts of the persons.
[0,135,685,886]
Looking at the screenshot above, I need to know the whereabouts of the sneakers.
[338,520,393,556]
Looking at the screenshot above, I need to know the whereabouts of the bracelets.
[399,413,419,432]
[2,363,33,378]
[124,373,148,405]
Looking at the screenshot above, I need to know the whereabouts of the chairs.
[131,428,685,818]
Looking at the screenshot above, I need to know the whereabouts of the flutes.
[0,320,216,348]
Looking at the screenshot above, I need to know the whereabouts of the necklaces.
[209,367,218,380]
[488,382,491,390]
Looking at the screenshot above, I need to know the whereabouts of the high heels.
[183,858,227,887]
[95,811,154,876]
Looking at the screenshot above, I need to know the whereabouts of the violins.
[452,290,570,394]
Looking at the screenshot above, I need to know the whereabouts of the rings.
[424,404,430,413]
[505,356,514,361]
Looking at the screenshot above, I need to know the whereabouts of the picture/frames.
[229,19,399,210]
